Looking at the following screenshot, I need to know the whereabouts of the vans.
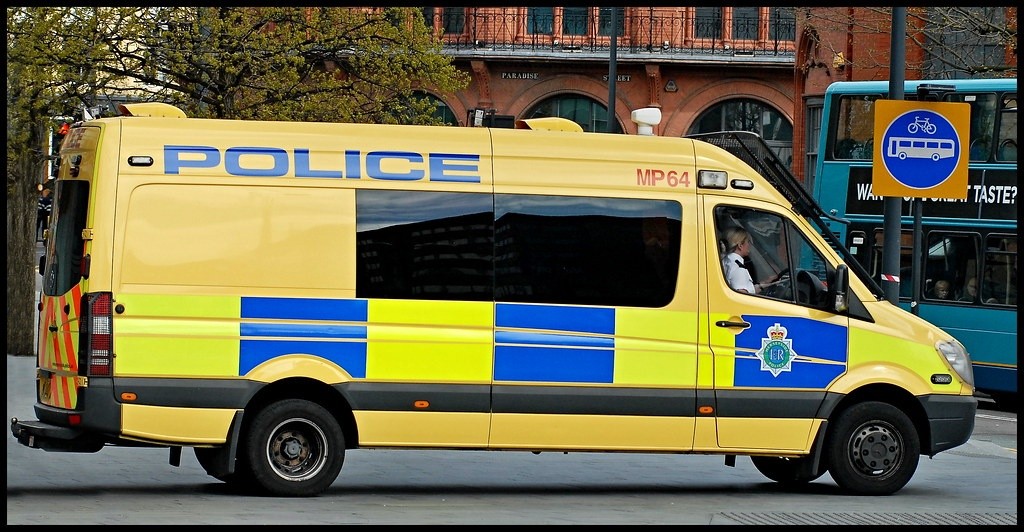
[12,103,976,497]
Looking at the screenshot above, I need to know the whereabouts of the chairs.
[969,138,987,161]
[999,139,1017,161]
[863,137,873,159]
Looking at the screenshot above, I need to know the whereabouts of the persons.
[36,188,52,240]
[722,226,782,296]
[928,276,987,301]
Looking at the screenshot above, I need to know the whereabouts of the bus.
[811,79,1019,412]
